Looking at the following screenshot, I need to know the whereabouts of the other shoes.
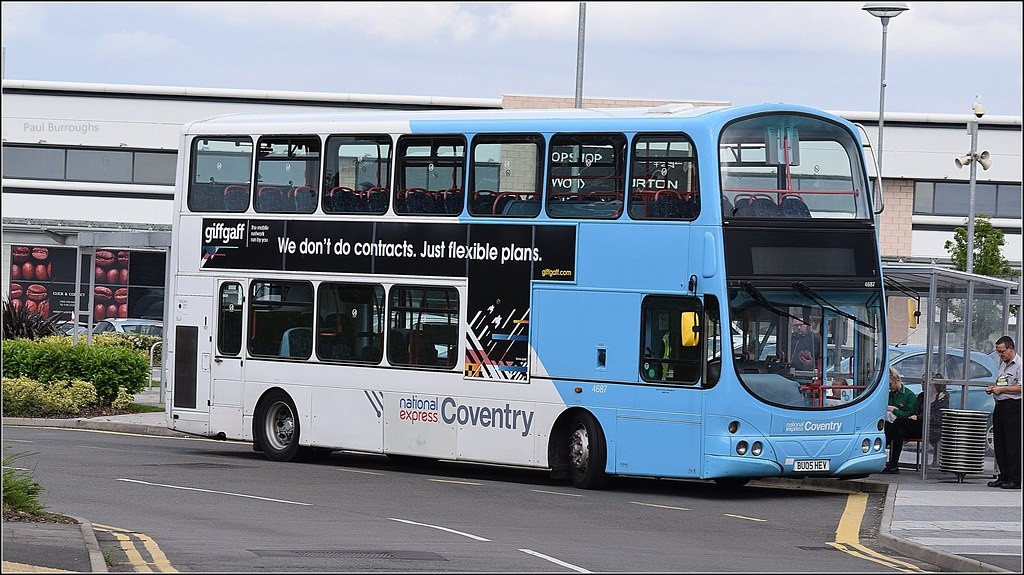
[987,477,1021,489]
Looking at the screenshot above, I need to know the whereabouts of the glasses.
[997,347,1011,353]
[794,323,804,327]
[921,379,926,385]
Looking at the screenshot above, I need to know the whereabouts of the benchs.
[903,438,937,471]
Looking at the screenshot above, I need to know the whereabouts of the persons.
[651,311,696,381]
[879,365,950,475]
[832,378,848,399]
[985,336,1022,489]
[791,318,821,371]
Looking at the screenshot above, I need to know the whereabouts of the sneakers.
[881,464,899,474]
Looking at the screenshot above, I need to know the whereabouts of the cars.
[90,318,164,365]
[808,343,1000,455]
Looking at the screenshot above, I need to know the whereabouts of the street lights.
[861,0,908,251]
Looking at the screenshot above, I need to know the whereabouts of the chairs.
[659,331,679,380]
[227,313,458,367]
[225,185,813,219]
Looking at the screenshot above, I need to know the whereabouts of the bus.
[164,101,922,490]
